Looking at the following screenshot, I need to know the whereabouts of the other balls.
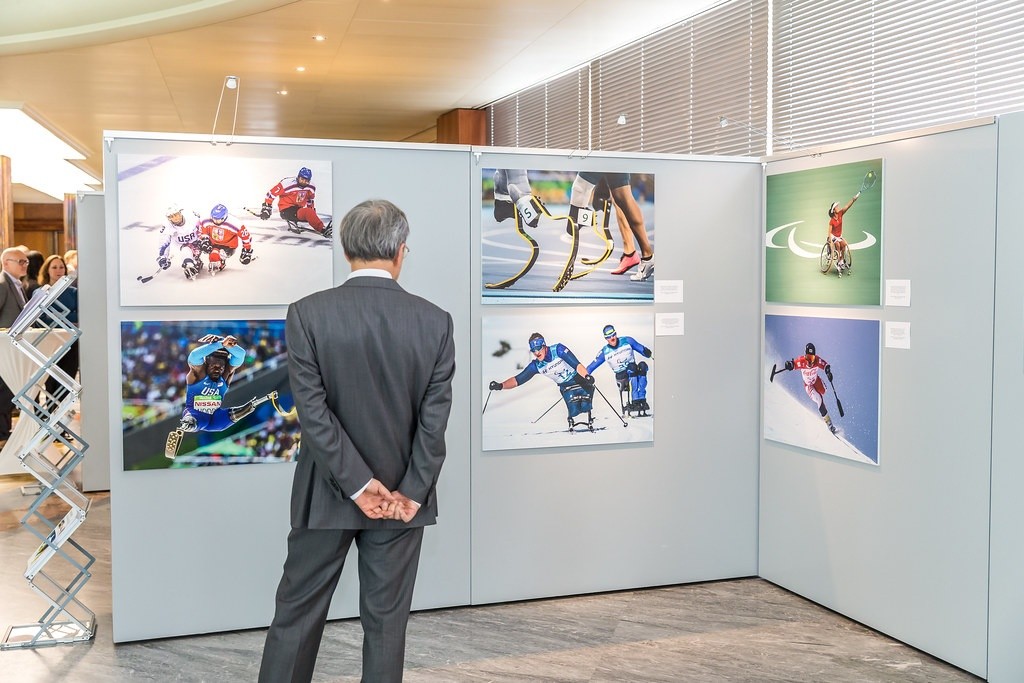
[137,276,142,281]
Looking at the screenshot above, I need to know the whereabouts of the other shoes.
[632,399,650,412]
[837,259,846,271]
[54,435,73,443]
[11,408,20,416]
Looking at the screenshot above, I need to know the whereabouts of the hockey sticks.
[243,206,262,218]
[141,244,187,283]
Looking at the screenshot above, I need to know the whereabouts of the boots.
[822,412,836,432]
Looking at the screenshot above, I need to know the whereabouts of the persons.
[827,191,861,270]
[0,245,79,451]
[785,343,836,432]
[489,333,595,417]
[604,173,654,281]
[485,169,615,292]
[156,204,212,279]
[258,200,457,683]
[260,167,333,237]
[182,204,254,280]
[586,325,652,410]
[165,334,279,460]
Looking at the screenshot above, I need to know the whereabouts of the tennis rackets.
[853,170,877,200]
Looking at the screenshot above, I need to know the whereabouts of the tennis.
[868,172,873,178]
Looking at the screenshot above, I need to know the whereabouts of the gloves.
[489,381,503,390]
[825,365,833,382]
[785,361,794,371]
[239,249,253,263]
[201,237,212,254]
[261,203,272,220]
[156,256,172,270]
[643,348,651,358]
[585,374,594,384]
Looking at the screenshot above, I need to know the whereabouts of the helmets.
[164,202,182,217]
[211,204,229,220]
[299,168,312,180]
[806,343,815,355]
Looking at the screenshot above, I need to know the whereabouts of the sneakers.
[610,250,641,275]
[629,254,654,282]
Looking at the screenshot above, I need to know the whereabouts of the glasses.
[530,345,543,353]
[606,332,615,339]
[403,245,411,258]
[6,258,29,267]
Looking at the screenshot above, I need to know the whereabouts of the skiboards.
[824,425,844,434]
